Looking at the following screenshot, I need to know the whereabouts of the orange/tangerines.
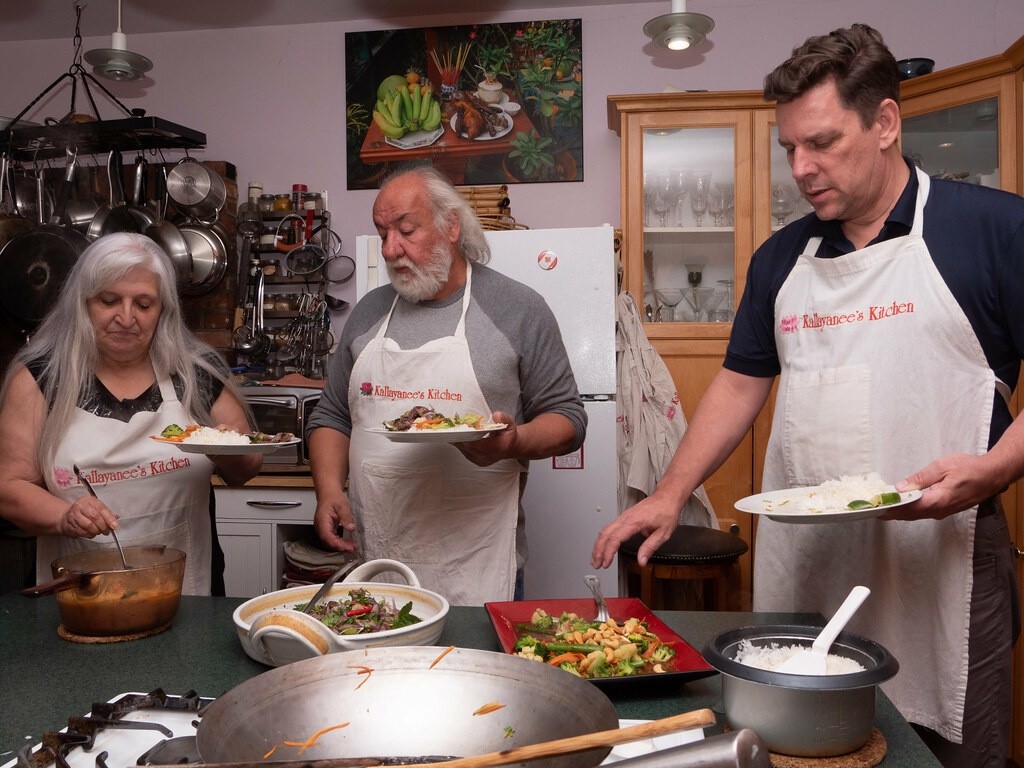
[406,72,434,101]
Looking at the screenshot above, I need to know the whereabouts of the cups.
[797,194,815,218]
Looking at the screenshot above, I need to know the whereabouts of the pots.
[0,149,234,359]
[20,543,186,636]
[232,557,450,665]
[196,645,769,768]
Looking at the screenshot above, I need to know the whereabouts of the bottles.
[263,294,276,311]
[247,181,263,212]
[291,292,301,309]
[250,258,261,276]
[303,192,322,210]
[274,194,292,212]
[262,259,282,277]
[289,220,301,243]
[291,184,307,210]
[259,194,275,211]
[278,227,290,244]
[259,226,278,244]
[275,294,291,311]
[263,326,275,345]
[275,327,290,346]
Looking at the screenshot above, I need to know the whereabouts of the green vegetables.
[418,413,484,428]
[294,589,422,635]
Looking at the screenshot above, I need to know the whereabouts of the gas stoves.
[0,688,704,768]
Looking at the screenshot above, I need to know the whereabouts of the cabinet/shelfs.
[607,35,1024,612]
[235,209,331,378]
[209,475,350,597]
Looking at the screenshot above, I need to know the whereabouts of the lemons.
[868,492,901,507]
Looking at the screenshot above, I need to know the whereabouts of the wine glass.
[770,184,795,226]
[642,167,735,229]
[642,263,734,323]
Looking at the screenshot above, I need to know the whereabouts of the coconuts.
[378,75,408,101]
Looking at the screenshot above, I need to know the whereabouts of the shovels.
[782,585,871,675]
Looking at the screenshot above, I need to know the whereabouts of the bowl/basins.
[701,623,900,757]
[504,102,521,116]
[896,57,935,79]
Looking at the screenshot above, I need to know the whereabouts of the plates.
[362,424,507,442]
[146,431,302,455]
[484,597,720,700]
[472,91,510,105]
[733,485,923,523]
[449,111,514,140]
[384,122,445,150]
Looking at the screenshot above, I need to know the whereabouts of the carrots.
[149,425,198,442]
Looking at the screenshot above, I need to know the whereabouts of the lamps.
[643,0,715,52]
[84,0,154,81]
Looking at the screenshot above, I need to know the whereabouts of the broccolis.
[161,424,184,437]
[512,610,675,678]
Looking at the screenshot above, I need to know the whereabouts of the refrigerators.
[355,223,619,599]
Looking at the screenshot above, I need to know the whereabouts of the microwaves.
[239,385,325,465]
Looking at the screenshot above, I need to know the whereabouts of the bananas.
[373,84,442,138]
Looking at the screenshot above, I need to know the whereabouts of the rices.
[796,472,893,511]
[182,426,251,446]
[729,638,868,676]
[408,423,478,432]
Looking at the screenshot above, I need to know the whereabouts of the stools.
[619,525,749,612]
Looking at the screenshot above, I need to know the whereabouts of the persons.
[303,167,587,607]
[0,233,263,597]
[591,24,1024,768]
